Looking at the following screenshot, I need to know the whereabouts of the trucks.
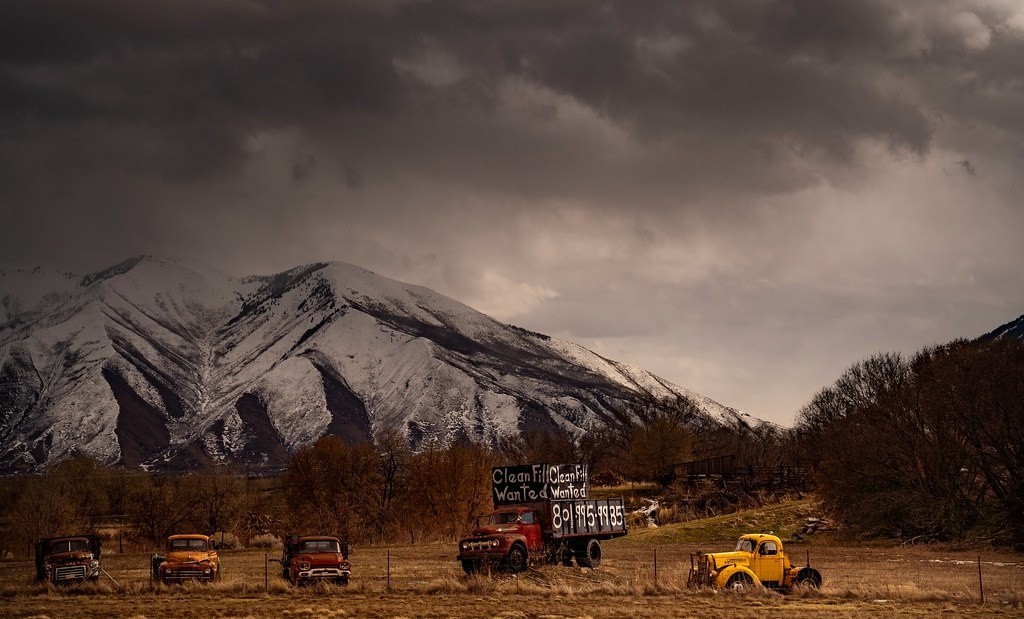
[687,533,823,597]
[152,535,222,588]
[281,532,351,587]
[33,533,102,588]
[456,461,630,577]
